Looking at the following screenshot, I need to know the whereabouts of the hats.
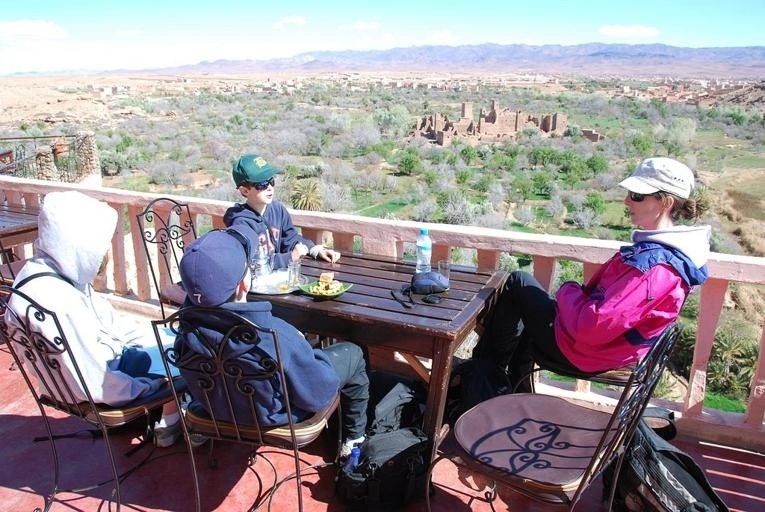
[618,157,695,202]
[232,153,285,188]
[180,224,260,308]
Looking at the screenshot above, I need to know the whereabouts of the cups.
[248,247,302,292]
[437,260,450,280]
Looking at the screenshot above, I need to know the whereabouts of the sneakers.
[184,428,210,448]
[152,414,183,448]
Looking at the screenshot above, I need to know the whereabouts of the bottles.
[414,228,432,274]
[341,448,361,473]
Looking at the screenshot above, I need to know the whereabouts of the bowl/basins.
[296,279,354,300]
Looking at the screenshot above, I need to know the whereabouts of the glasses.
[248,177,274,190]
[631,192,657,202]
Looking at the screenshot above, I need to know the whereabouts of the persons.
[172,223,371,464]
[471,153,715,397]
[222,153,343,270]
[4,187,210,451]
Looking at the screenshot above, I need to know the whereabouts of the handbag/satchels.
[336,429,435,510]
[445,359,512,428]
[366,380,421,430]
[121,347,152,381]
